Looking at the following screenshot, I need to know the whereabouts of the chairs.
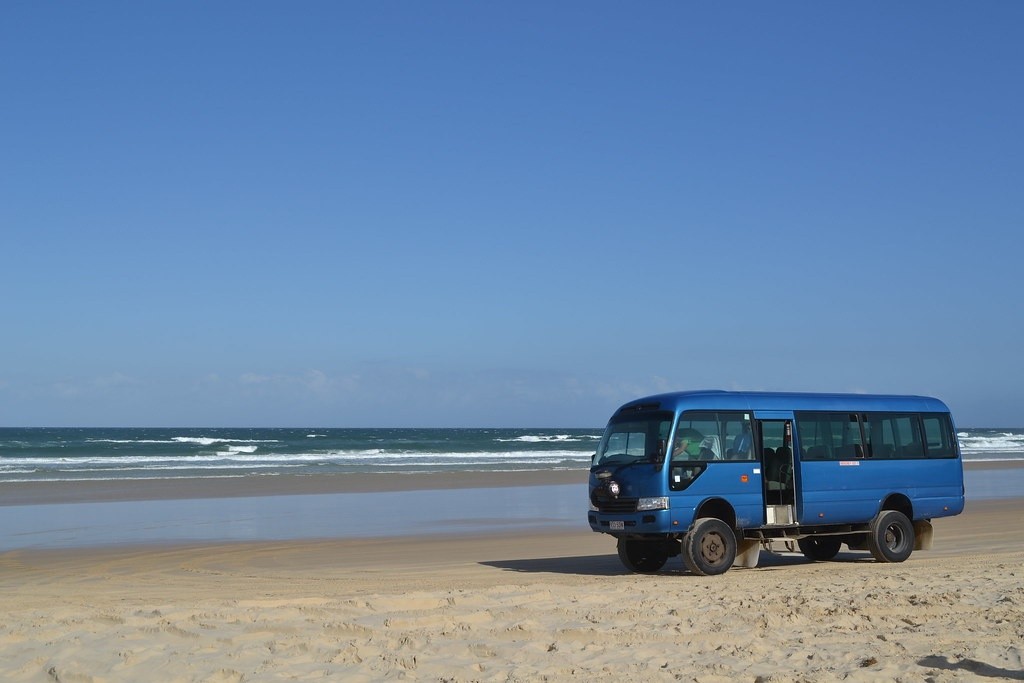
[646,436,942,505]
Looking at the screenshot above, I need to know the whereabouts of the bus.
[587,388,965,576]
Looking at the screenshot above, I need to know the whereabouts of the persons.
[672,428,704,459]
